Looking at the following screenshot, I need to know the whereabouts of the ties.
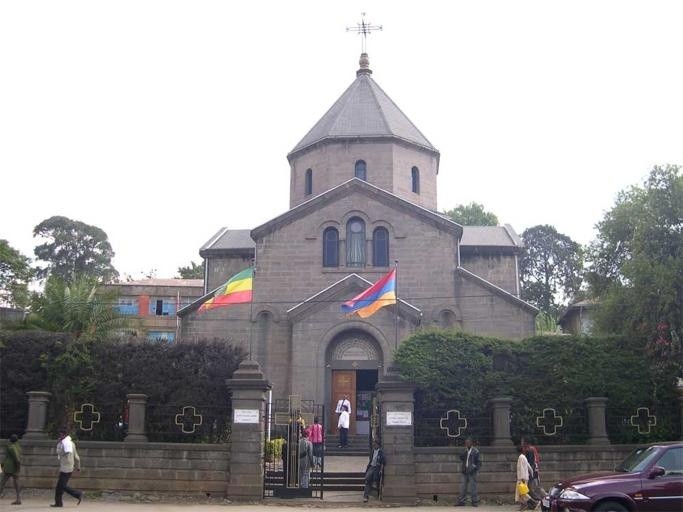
[339,399,345,411]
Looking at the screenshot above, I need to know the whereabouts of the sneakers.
[473,502,478,507]
[363,496,368,502]
[11,502,22,505]
[454,503,464,506]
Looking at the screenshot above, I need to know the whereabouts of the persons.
[50,427,84,507]
[0,433,22,505]
[281,393,386,502]
[453,437,481,507]
[514,435,549,512]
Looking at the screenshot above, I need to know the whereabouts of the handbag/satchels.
[517,480,529,496]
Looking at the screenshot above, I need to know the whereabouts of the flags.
[176,266,252,320]
[340,264,397,319]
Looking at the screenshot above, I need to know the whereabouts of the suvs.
[540,441,682,512]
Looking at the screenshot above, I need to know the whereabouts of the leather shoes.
[77,492,82,505]
[50,504,63,507]
